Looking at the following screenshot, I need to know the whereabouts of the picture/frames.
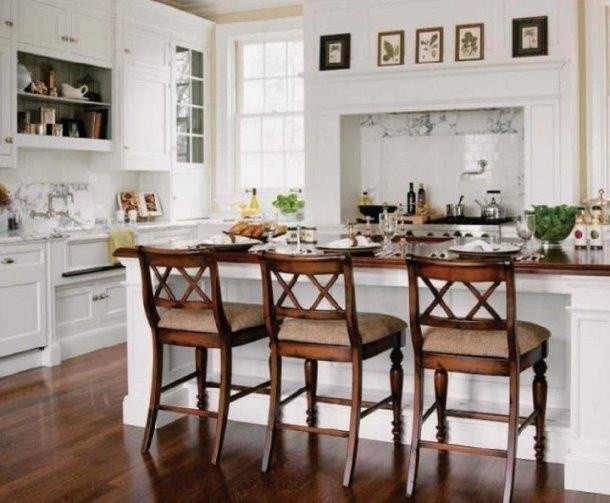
[376,28,406,67]
[413,25,444,64]
[317,32,353,70]
[454,22,485,61]
[510,14,550,58]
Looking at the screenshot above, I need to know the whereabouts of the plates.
[444,243,521,256]
[311,243,382,254]
[194,233,262,252]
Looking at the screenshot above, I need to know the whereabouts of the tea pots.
[474,187,506,219]
[60,81,89,98]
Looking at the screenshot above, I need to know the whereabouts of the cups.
[116,210,124,228]
[129,209,137,225]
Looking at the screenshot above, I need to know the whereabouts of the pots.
[446,194,465,216]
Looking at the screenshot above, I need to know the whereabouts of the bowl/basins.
[356,204,397,219]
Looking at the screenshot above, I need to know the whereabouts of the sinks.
[34,222,111,233]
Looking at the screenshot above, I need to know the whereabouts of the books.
[75,107,109,140]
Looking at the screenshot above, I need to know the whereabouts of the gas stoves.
[356,215,511,237]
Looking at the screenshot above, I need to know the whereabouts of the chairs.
[394,249,556,502]
[131,236,287,469]
[254,243,404,486]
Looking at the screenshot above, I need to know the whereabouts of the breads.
[228,222,264,238]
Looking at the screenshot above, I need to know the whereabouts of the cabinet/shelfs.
[1,0,18,173]
[131,225,200,240]
[0,235,54,377]
[140,8,216,222]
[15,1,116,68]
[196,220,233,237]
[114,0,178,174]
[49,224,130,364]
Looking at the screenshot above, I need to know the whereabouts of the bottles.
[248,187,259,209]
[407,181,425,216]
[571,205,604,250]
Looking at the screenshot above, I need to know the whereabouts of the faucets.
[48,192,74,209]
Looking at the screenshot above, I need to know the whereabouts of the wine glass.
[377,213,396,257]
[261,209,280,247]
[513,213,536,260]
